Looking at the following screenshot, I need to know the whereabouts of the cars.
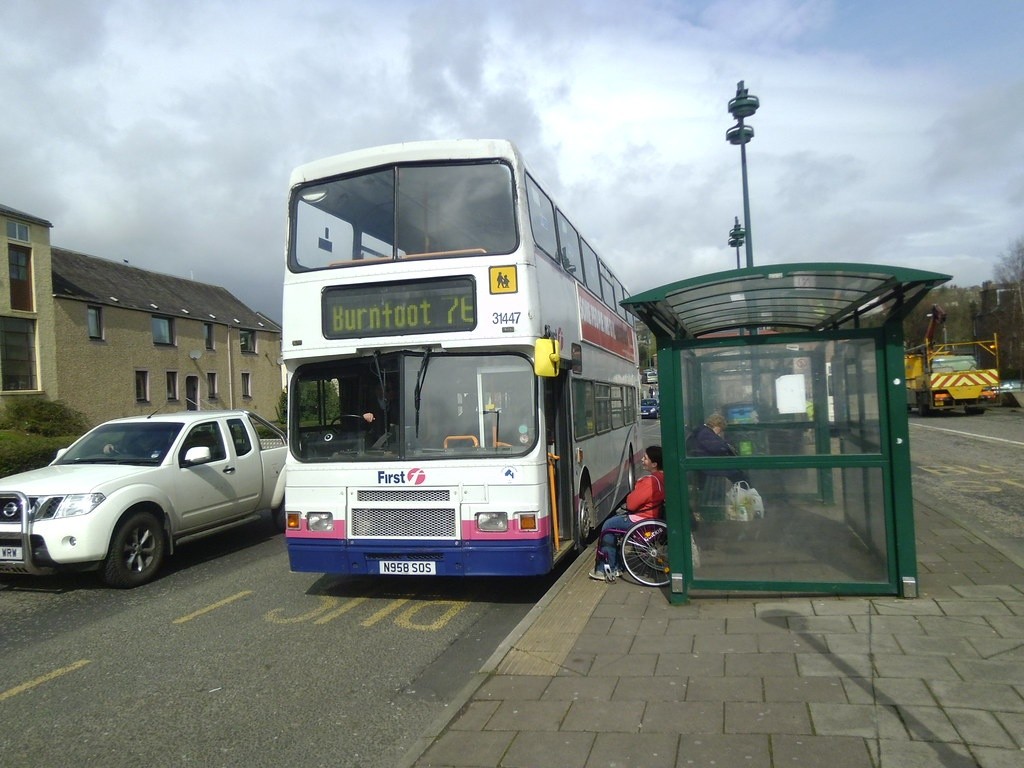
[641,369,659,383]
[638,398,661,419]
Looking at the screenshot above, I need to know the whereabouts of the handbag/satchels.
[725,480,765,521]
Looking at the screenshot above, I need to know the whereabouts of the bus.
[279,136,644,580]
[808,359,879,432]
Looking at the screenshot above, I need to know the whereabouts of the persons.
[686,413,752,494]
[648,385,654,399]
[588,445,664,580]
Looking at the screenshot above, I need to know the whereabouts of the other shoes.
[589,568,624,580]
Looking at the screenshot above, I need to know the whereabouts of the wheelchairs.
[595,500,670,587]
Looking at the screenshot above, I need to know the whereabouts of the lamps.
[189,349,202,361]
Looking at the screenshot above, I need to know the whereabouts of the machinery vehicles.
[903,303,999,418]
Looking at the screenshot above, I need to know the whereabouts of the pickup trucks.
[0,409,286,589]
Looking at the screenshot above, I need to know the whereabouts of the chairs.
[188,430,218,461]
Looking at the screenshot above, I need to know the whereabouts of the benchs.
[699,475,728,522]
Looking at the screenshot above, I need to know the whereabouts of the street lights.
[726,79,762,407]
